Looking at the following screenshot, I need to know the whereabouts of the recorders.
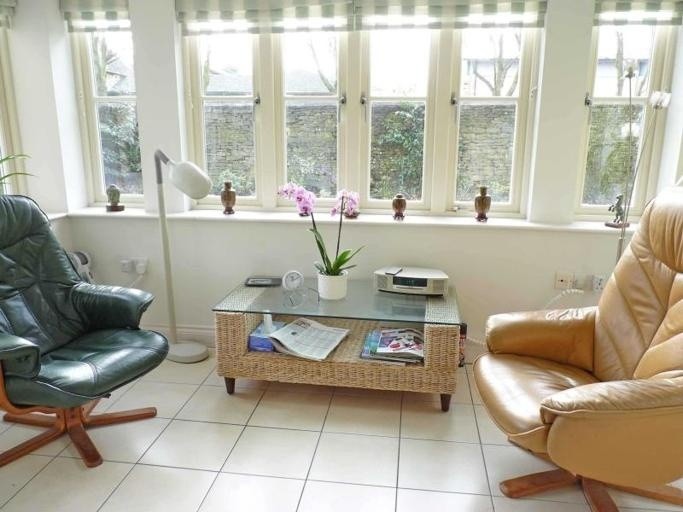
[374,266,449,296]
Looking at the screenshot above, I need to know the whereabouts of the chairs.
[0,195,168,470]
[467,173,682,511]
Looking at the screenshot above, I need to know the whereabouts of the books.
[267,316,350,362]
[359,325,425,368]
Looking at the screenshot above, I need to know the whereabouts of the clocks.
[283,270,303,292]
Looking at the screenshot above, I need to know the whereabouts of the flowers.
[276,180,364,274]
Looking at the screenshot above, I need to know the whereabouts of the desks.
[211,275,463,412]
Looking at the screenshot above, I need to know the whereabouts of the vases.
[316,272,348,300]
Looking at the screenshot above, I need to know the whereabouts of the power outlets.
[131,255,148,274]
[593,276,605,292]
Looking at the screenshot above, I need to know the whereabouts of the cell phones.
[385,267,402,275]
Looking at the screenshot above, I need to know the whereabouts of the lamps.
[609,44,673,267]
[153,148,211,364]
